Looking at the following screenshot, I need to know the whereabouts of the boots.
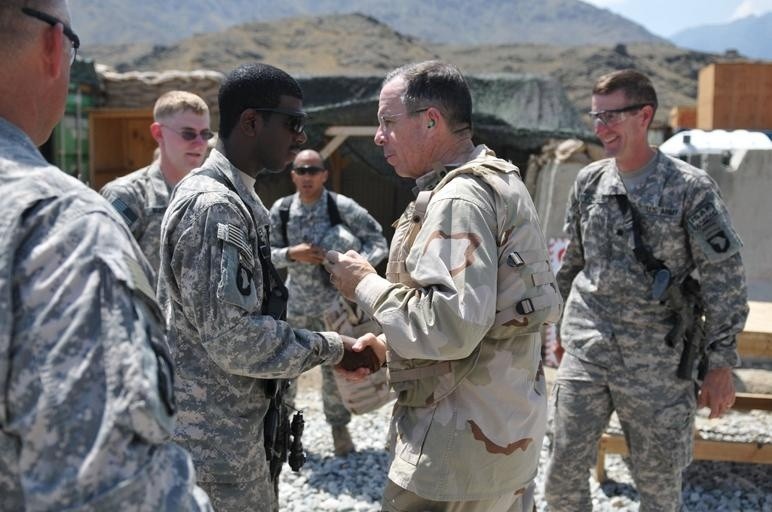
[332,426,354,456]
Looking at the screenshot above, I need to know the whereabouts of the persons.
[545,69,752,512]
[268,151,388,455]
[1,0,214,512]
[99,91,216,295]
[158,61,381,512]
[324,58,561,512]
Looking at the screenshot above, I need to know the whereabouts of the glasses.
[378,107,429,130]
[19,4,79,69]
[292,166,326,176]
[589,103,647,127]
[252,106,313,134]
[161,124,214,141]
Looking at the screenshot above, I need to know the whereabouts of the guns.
[265,284,305,482]
[651,269,710,382]
